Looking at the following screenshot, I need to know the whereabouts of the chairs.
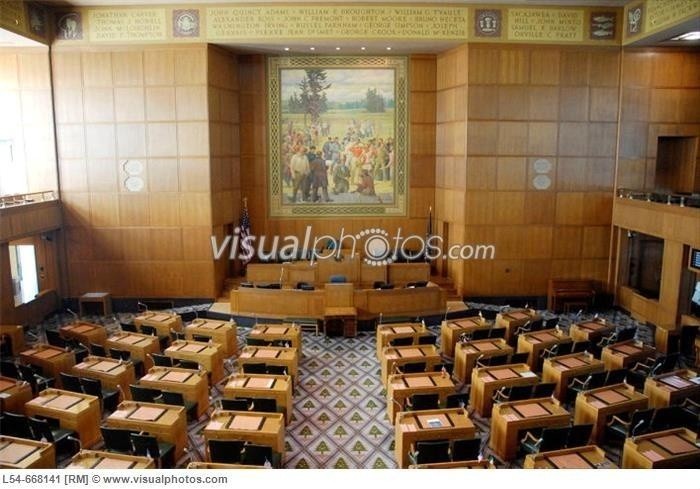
[0,190,699,466]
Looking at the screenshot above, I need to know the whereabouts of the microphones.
[27,301,258,468]
[379,304,662,469]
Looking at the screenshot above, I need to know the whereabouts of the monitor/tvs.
[270,283,280,289]
[302,286,315,290]
[380,284,395,289]
[690,248,700,270]
[415,282,427,287]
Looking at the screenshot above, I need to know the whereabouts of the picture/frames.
[263,53,408,219]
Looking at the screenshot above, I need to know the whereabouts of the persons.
[279,118,396,203]
